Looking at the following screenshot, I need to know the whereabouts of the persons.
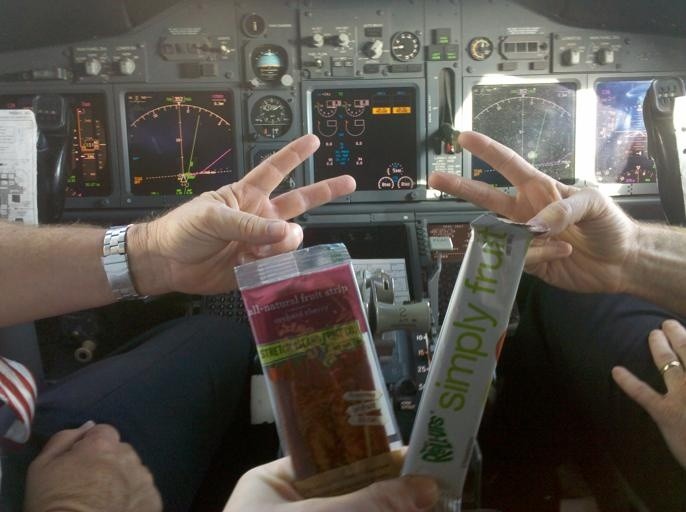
[221,443,443,512]
[427,129,685,511]
[1,133,358,509]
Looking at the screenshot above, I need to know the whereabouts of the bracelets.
[99,221,147,304]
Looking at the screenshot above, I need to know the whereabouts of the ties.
[0,358,39,450]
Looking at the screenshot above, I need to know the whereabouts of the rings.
[660,360,682,375]
